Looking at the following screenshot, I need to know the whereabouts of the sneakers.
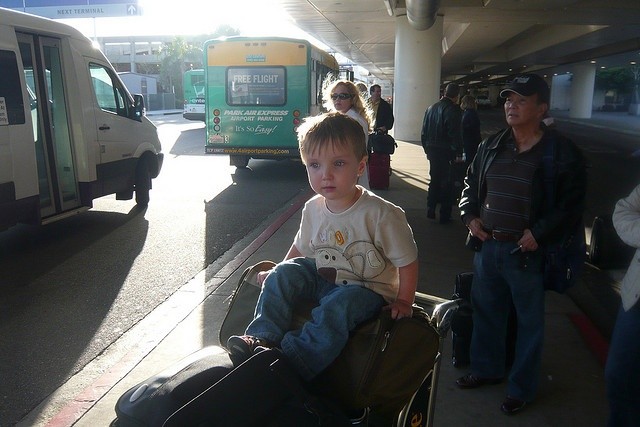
[227,335,268,362]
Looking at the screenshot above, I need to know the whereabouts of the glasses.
[330,94,356,100]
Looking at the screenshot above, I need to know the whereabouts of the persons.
[226,111,420,381]
[329,80,375,191]
[356,82,368,100]
[366,84,394,135]
[421,83,464,224]
[460,94,483,177]
[455,72,588,415]
[603,184,640,427]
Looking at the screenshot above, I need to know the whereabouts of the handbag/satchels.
[218,261,440,411]
[366,128,399,154]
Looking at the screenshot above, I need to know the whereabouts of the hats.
[499,73,549,106]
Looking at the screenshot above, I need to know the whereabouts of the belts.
[480,228,524,243]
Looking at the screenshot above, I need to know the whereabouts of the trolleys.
[111,260,462,427]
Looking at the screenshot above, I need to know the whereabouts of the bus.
[183,69,205,119]
[205,36,354,166]
[0,6,164,229]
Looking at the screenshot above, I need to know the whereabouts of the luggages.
[450,272,514,367]
[366,127,392,191]
[114,345,350,425]
[588,215,636,268]
